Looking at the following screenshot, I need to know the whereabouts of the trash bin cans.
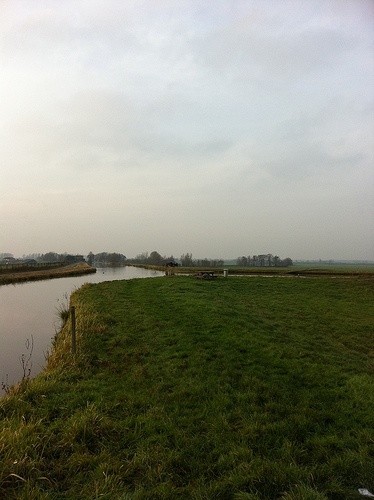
[223,269,228,278]
